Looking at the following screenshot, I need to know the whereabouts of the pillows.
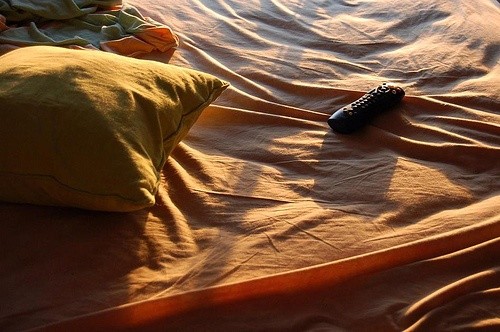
[1,45,230,212]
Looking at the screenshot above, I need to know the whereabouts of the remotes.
[328,83,405,133]
